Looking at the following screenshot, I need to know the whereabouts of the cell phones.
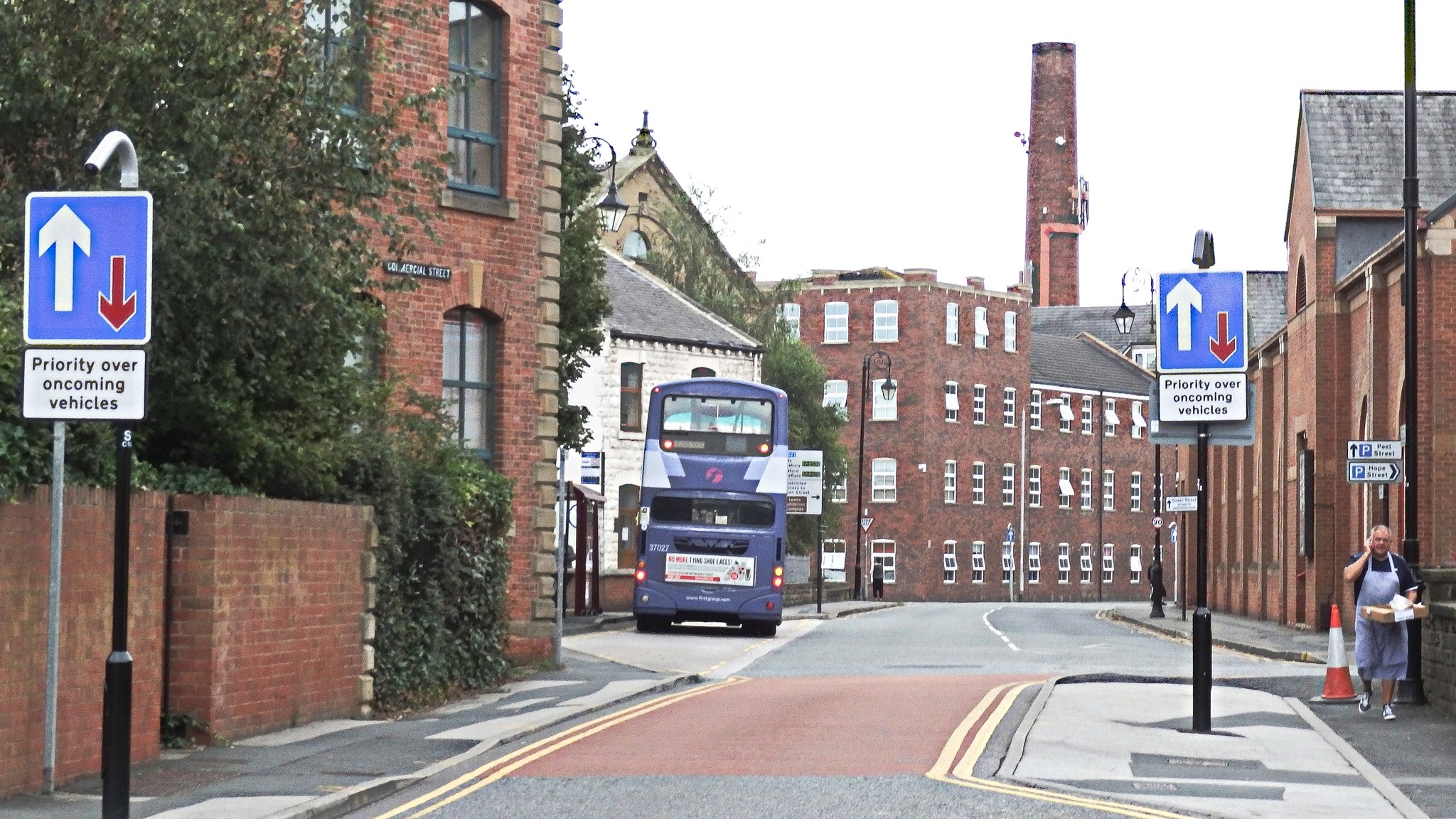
[1370,538,1373,543]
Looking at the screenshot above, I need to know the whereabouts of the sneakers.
[1382,702,1396,720]
[1358,690,1372,714]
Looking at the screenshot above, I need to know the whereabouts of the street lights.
[1112,267,1168,618]
[852,350,899,599]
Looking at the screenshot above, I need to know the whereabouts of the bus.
[631,376,789,637]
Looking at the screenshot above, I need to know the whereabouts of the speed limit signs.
[1151,517,1164,529]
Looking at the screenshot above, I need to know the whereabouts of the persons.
[554,535,575,608]
[1344,526,1417,721]
[873,557,883,601]
[1147,560,1166,605]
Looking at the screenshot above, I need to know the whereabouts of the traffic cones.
[1307,602,1364,704]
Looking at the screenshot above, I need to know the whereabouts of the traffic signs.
[1165,495,1198,513]
[1346,462,1403,484]
[1346,440,1402,460]
